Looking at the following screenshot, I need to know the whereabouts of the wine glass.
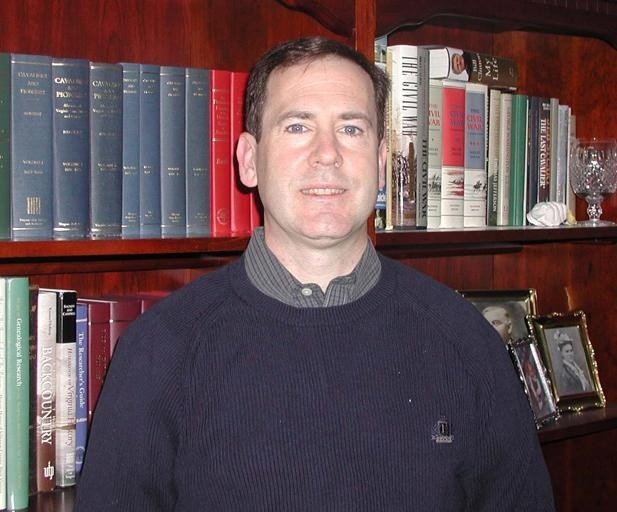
[569,142,617,228]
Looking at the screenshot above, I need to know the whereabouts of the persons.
[70,34,556,512]
[552,329,592,394]
[522,357,550,417]
[481,305,515,349]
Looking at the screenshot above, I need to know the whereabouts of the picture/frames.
[454,288,606,430]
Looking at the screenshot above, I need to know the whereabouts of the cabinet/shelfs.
[0,0,617,512]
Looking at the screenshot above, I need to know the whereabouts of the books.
[0,276,161,512]
[375,36,578,230]
[2,53,263,241]
